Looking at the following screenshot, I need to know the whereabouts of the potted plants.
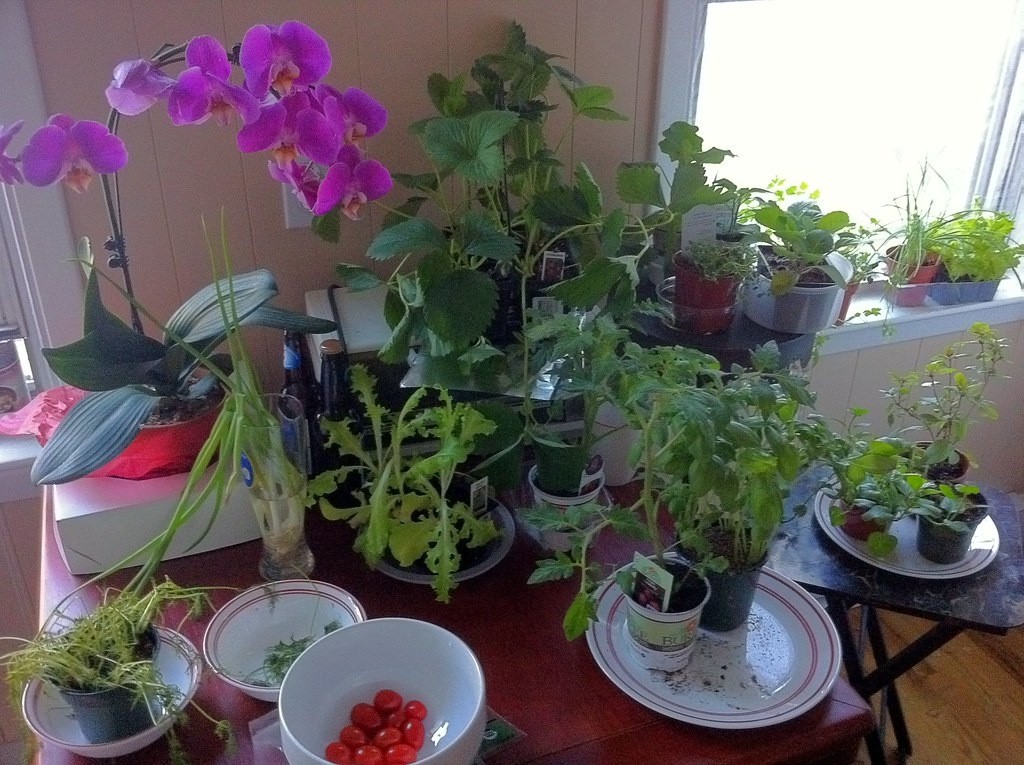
[0,22,1023,747]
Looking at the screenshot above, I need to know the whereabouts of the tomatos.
[326,689,428,765]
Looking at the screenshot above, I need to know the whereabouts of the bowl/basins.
[20,623,203,757]
[203,578,367,702]
[280,617,485,765]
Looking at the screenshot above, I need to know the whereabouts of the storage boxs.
[53,459,260,575]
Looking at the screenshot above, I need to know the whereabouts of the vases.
[115,366,228,477]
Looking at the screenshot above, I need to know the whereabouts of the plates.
[583,553,842,729]
[816,477,999,579]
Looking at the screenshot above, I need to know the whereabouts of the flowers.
[1,21,393,487]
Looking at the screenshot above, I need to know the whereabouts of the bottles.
[312,337,361,500]
[280,330,321,477]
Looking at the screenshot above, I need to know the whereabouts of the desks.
[763,462,1024,765]
[33,471,877,765]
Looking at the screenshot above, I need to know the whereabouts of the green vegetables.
[217,616,342,688]
